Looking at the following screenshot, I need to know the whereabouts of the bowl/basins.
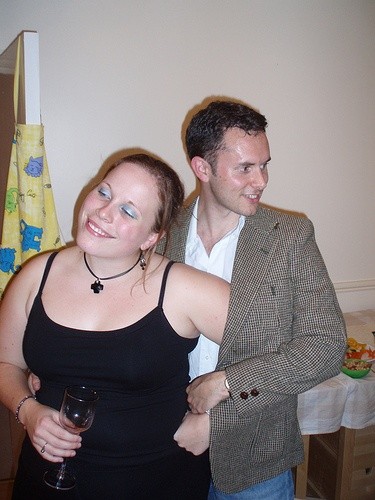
[342,358,373,379]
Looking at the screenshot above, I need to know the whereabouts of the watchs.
[225,378,230,390]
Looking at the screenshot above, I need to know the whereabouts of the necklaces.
[83,250,143,293]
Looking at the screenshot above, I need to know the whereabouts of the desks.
[294,308,375,500]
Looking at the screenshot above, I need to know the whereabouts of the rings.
[41,443,48,453]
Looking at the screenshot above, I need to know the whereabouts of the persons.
[15,395,36,425]
[0,147,231,500]
[153,99,348,499]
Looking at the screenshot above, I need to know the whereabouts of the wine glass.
[42,385,100,490]
